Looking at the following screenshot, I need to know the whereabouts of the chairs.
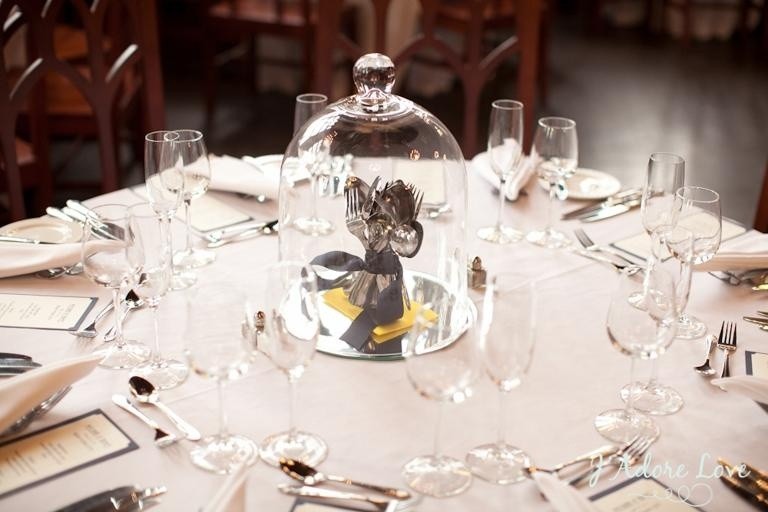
[0,1,550,221]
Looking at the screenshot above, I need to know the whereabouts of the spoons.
[0,229,85,279]
[125,374,201,442]
[695,329,718,378]
[45,199,128,252]
[340,178,432,314]
[570,245,643,279]
[275,457,413,502]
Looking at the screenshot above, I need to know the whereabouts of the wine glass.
[528,115,579,252]
[594,153,724,450]
[475,98,528,245]
[77,127,212,391]
[401,246,539,497]
[179,261,334,473]
[292,89,335,239]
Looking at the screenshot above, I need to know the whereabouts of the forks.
[111,395,187,450]
[567,431,659,489]
[714,320,738,378]
[574,225,648,275]
